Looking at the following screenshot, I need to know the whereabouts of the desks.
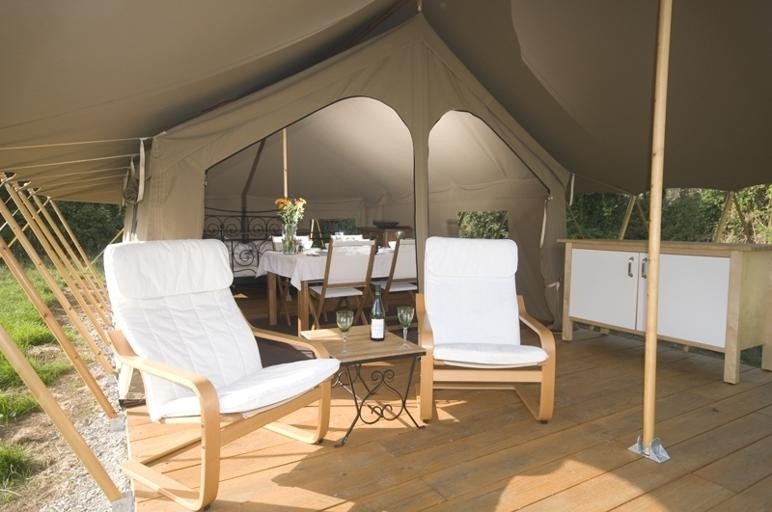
[296,324,425,448]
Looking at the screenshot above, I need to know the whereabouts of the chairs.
[260,234,416,327]
[104,239,338,512]
[415,234,554,423]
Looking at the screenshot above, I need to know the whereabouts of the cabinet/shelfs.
[556,239,771,384]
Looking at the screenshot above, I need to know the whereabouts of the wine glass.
[335,310,353,357]
[396,306,414,352]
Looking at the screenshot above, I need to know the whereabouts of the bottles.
[370,284,386,341]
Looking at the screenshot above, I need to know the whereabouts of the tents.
[122,13,572,334]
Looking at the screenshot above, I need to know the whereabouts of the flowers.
[273,197,307,250]
